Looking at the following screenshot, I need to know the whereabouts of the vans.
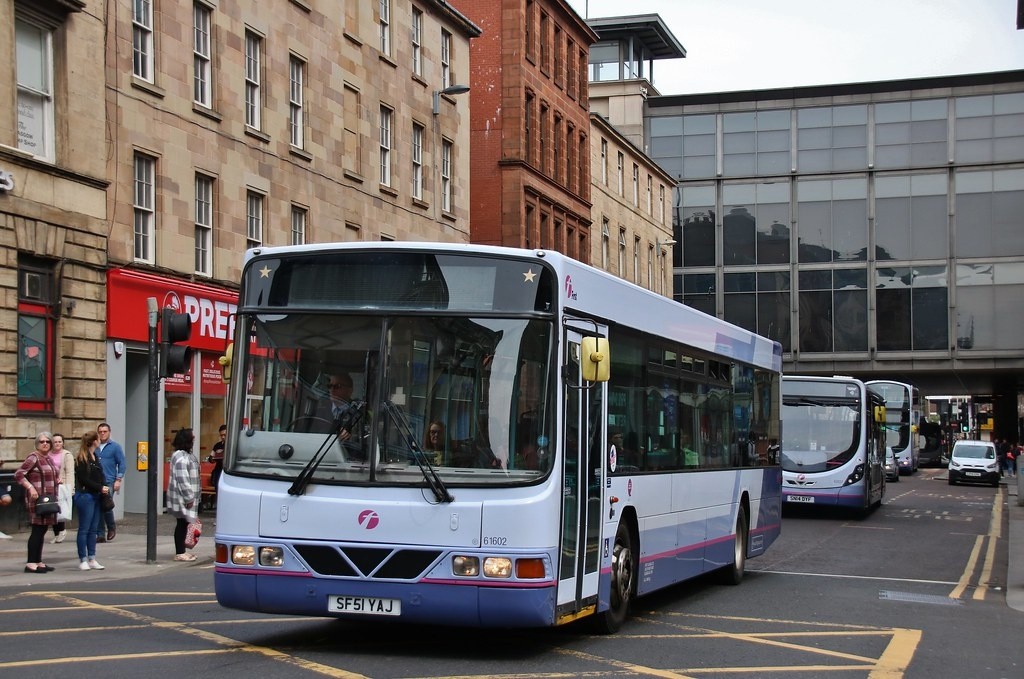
[945,440,1005,488]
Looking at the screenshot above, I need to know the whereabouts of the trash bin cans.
[1016,452,1024,507]
[0,459,38,536]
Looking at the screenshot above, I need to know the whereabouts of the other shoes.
[48,535,58,544]
[78,560,90,571]
[173,552,197,561]
[88,561,105,570]
[25,566,46,573]
[39,565,54,571]
[56,529,67,543]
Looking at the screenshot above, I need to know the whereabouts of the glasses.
[327,384,350,390]
[39,441,50,444]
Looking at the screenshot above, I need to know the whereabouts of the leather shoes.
[96,537,106,543]
[107,528,115,540]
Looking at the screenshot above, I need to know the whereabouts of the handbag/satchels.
[101,491,115,512]
[184,506,202,549]
[34,493,59,516]
[1007,451,1015,461]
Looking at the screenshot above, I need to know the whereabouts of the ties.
[334,408,341,420]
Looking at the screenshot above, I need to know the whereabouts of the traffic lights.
[160,308,192,379]
[958,403,967,426]
[961,415,970,433]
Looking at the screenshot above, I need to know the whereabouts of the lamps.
[656,238,677,256]
[433,85,471,114]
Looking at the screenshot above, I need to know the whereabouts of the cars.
[886,446,900,482]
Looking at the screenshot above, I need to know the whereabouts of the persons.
[748,431,767,466]
[476,413,538,471]
[47,433,74,543]
[208,424,227,525]
[995,438,1020,480]
[681,433,699,465]
[424,420,455,465]
[95,423,126,543]
[618,431,643,466]
[74,431,109,570]
[14,432,63,573]
[294,373,367,459]
[767,438,779,463]
[608,429,622,452]
[166,428,201,561]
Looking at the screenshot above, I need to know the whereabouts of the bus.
[781,374,886,519]
[917,421,942,468]
[213,239,783,634]
[865,380,921,476]
